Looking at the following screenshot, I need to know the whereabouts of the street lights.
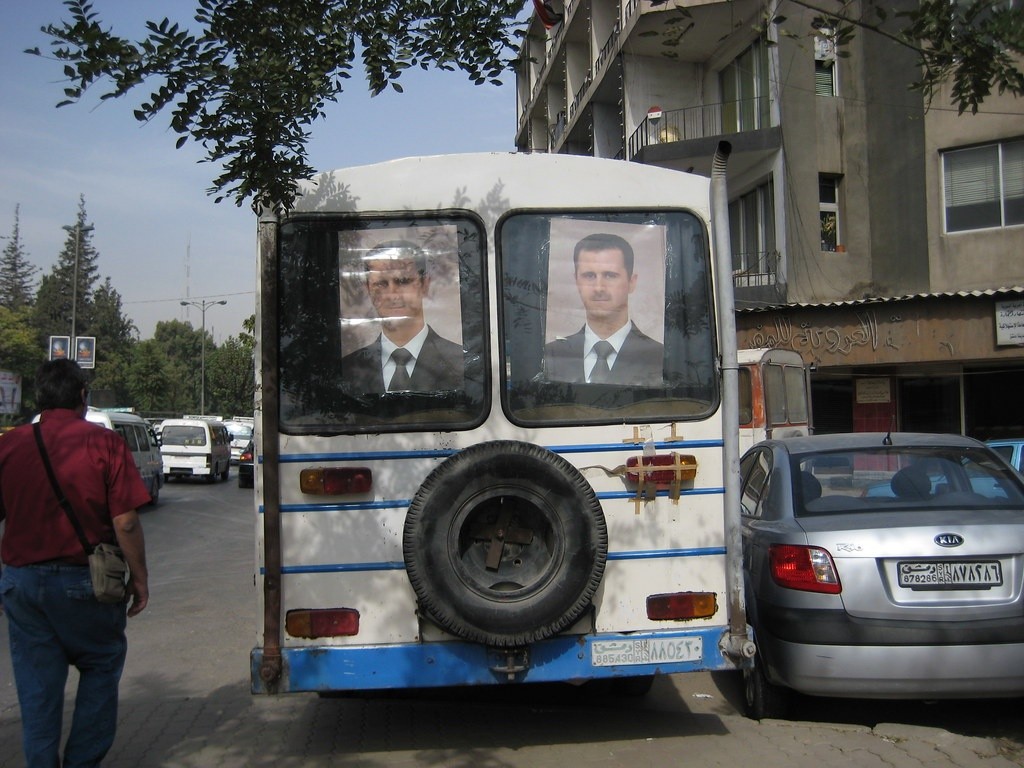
[62,224,95,360]
[180,300,228,415]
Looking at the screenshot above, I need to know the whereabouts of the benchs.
[804,491,991,512]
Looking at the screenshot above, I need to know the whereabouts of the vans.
[160,419,234,483]
[32,410,165,507]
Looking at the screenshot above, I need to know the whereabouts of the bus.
[249,144,755,695]
[736,347,829,456]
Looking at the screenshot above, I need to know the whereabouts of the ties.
[388,348,413,395]
[588,343,615,390]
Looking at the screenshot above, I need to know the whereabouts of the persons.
[545,233,664,388]
[0,358,151,768]
[342,240,463,391]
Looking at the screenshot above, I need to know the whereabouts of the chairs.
[801,471,822,506]
[890,466,935,499]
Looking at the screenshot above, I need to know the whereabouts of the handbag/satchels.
[89,544,130,604]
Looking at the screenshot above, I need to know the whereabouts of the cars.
[860,437,1024,502]
[739,415,1024,718]
[185,414,254,487]
